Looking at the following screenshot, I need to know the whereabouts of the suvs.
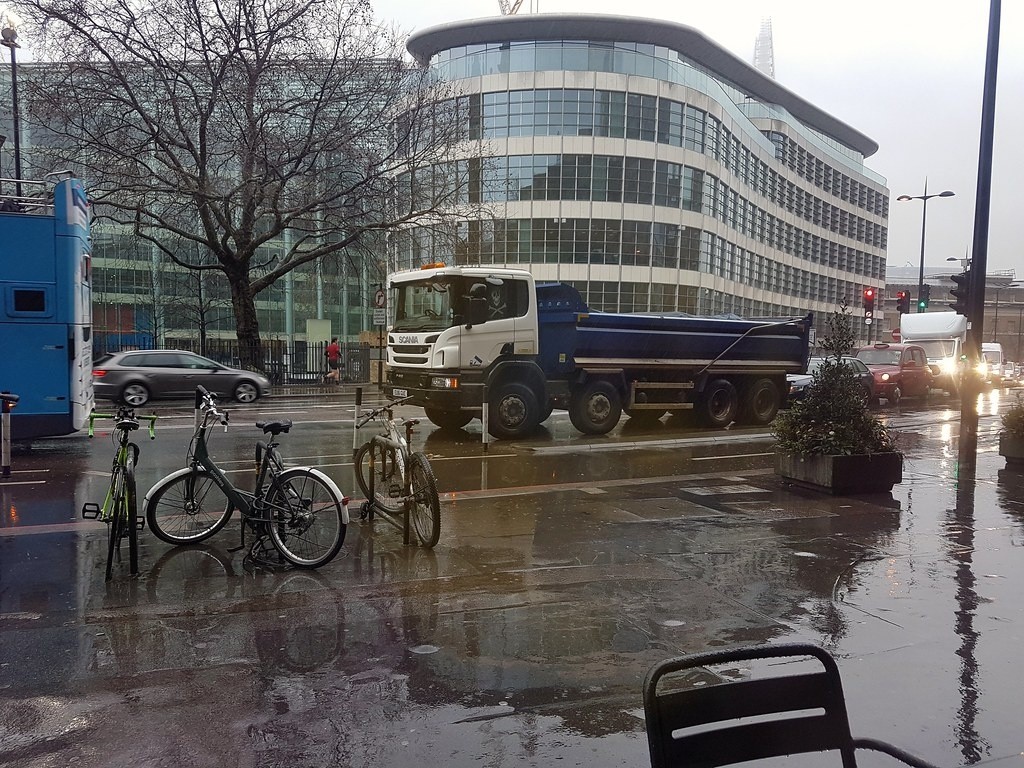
[90,351,272,402]
[784,355,875,409]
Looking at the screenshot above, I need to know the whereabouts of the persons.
[451,301,469,326]
[323,338,343,387]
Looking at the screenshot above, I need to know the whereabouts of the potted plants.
[998,390,1024,463]
[770,299,904,493]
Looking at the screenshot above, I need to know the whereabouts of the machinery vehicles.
[384,270,813,441]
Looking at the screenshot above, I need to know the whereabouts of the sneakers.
[335,380,339,385]
[325,376,328,385]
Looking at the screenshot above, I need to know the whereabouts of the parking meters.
[0,393,20,476]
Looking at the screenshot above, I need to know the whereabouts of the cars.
[1003,362,1024,386]
[979,352,988,381]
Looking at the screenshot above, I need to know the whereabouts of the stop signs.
[892,328,901,342]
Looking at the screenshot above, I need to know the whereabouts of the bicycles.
[143,391,351,571]
[346,394,442,548]
[89,409,159,581]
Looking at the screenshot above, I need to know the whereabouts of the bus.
[0,169,97,442]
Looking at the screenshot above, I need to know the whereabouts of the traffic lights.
[918,286,929,309]
[896,290,910,314]
[866,289,874,309]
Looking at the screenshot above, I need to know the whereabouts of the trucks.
[900,312,967,396]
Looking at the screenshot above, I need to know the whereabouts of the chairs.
[641,645,937,768]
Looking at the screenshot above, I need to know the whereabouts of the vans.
[981,342,1007,385]
[855,343,934,405]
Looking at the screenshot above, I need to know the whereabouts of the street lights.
[897,192,956,312]
[0,28,22,199]
[946,258,972,271]
[370,282,383,387]
[996,285,1020,342]
